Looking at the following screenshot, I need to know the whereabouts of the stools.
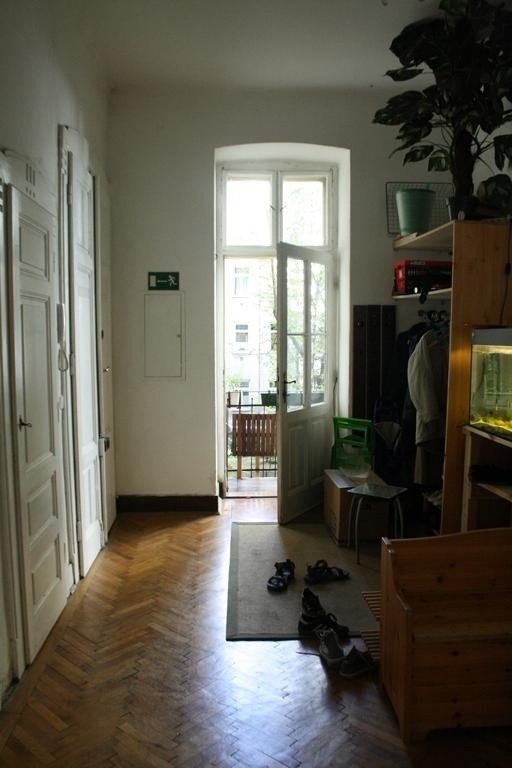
[345,481,409,564]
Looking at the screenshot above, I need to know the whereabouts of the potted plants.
[373,0,512,220]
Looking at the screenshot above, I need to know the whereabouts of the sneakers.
[297,588,365,678]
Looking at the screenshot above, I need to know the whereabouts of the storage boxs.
[324,465,389,547]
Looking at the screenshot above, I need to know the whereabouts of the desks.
[458,422,512,531]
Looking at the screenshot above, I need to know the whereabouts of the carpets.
[225,520,382,646]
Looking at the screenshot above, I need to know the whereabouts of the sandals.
[304,558,348,586]
[266,559,295,591]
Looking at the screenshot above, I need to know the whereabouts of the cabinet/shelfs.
[392,219,512,536]
[378,524,511,746]
